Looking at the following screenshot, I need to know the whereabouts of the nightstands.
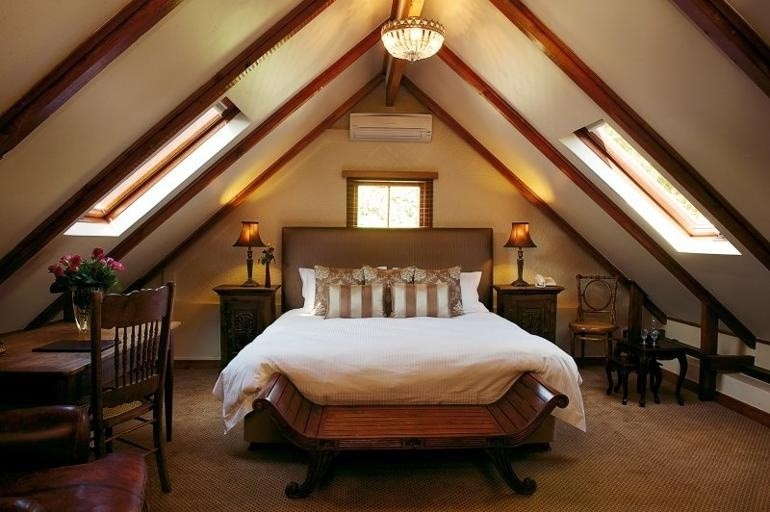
[493,283,566,343]
[211,284,281,381]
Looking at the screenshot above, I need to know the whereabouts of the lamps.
[232,221,268,288]
[380,15,447,63]
[502,219,539,287]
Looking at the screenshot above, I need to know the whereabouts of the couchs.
[1,404,149,512]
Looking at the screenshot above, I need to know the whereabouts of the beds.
[210,225,589,457]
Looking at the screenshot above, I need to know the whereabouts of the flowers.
[257,241,276,266]
[47,247,125,308]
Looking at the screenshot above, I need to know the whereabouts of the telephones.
[535,274,557,288]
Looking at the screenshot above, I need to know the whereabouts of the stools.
[605,356,663,405]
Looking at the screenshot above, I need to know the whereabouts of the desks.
[613,336,689,405]
[0,320,183,442]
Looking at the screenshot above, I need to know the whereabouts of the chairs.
[88,280,176,494]
[568,274,620,372]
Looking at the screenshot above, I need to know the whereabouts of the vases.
[71,288,92,332]
[264,261,271,289]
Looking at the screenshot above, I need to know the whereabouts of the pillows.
[392,266,489,316]
[414,264,467,317]
[362,264,414,317]
[312,264,365,316]
[324,280,392,318]
[389,279,454,318]
[296,264,388,314]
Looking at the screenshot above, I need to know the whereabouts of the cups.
[649,318,658,330]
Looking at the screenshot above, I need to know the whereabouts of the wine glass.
[640,328,650,346]
[649,331,659,347]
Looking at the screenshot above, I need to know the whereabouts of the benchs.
[251,371,568,498]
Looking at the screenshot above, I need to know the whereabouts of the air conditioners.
[349,112,433,144]
